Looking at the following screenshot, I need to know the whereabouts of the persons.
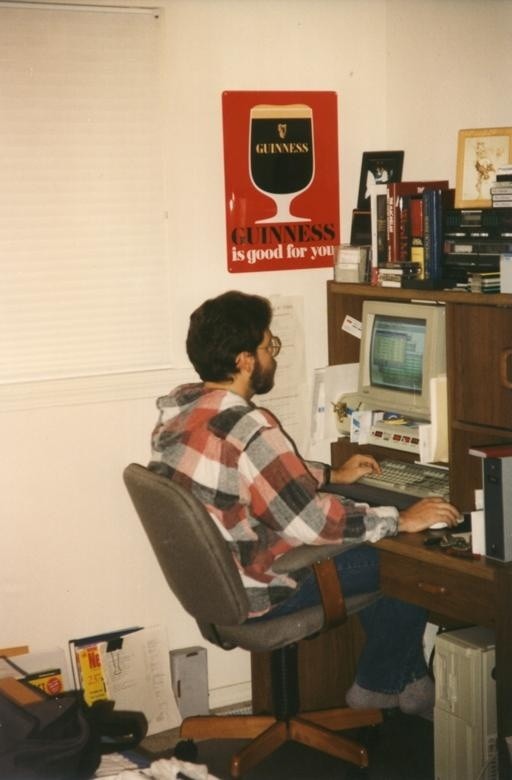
[149,291,462,717]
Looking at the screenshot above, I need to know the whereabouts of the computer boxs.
[432,626,501,780]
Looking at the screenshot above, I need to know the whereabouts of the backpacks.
[0,676,148,780]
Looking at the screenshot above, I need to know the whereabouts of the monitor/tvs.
[358,299,446,424]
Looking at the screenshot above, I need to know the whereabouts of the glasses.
[257,336,281,358]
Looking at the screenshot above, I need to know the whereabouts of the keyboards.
[357,458,450,503]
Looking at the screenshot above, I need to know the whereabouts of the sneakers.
[345,682,399,710]
[398,673,435,716]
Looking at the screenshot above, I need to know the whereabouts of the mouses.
[429,513,466,531]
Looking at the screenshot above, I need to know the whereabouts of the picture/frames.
[452,126,512,210]
[355,150,404,212]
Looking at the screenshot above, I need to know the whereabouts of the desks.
[251,282,511,763]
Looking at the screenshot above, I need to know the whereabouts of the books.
[365,172,512,296]
[67,623,146,708]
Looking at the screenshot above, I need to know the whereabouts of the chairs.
[122,464,385,779]
